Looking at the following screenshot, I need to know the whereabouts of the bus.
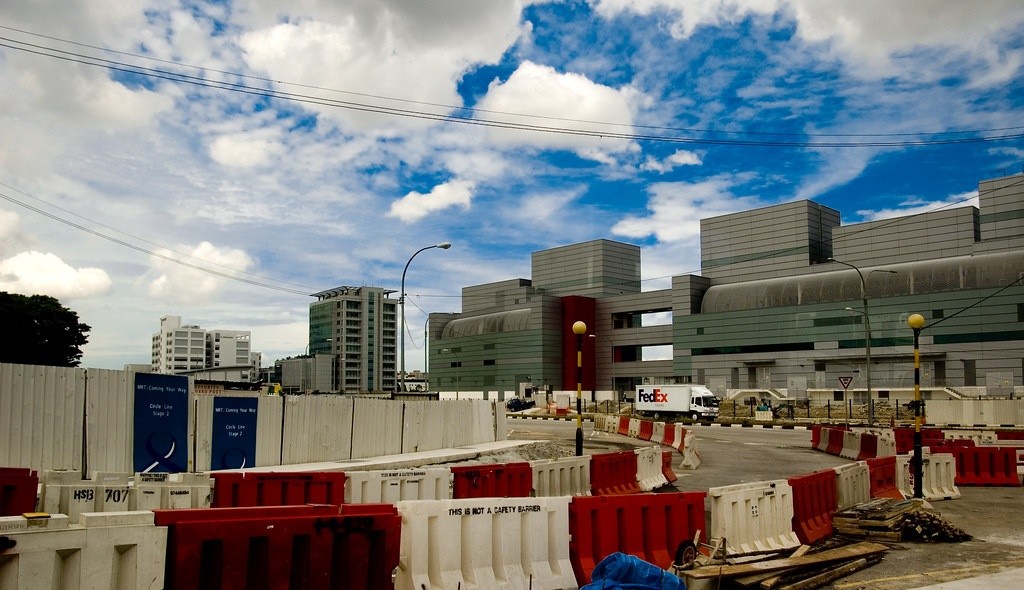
[396,378,429,393]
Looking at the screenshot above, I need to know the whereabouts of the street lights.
[908,313,925,501]
[589,334,616,416]
[826,257,898,425]
[572,320,587,456]
[305,338,333,355]
[400,242,452,391]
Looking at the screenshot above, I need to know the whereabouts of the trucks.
[636,383,721,422]
[506,397,536,412]
